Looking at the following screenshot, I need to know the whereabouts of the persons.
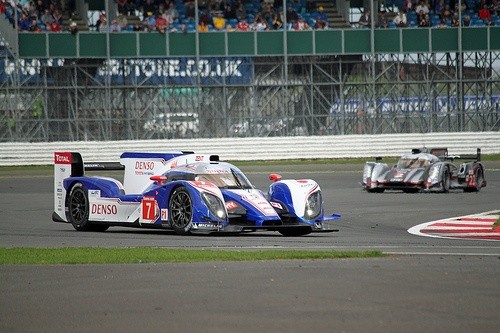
[1,0,500,29]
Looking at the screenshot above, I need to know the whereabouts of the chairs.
[387,0,500,28]
[122,0,327,31]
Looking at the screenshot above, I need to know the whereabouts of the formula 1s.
[363,146,487,193]
[52,151,341,236]
